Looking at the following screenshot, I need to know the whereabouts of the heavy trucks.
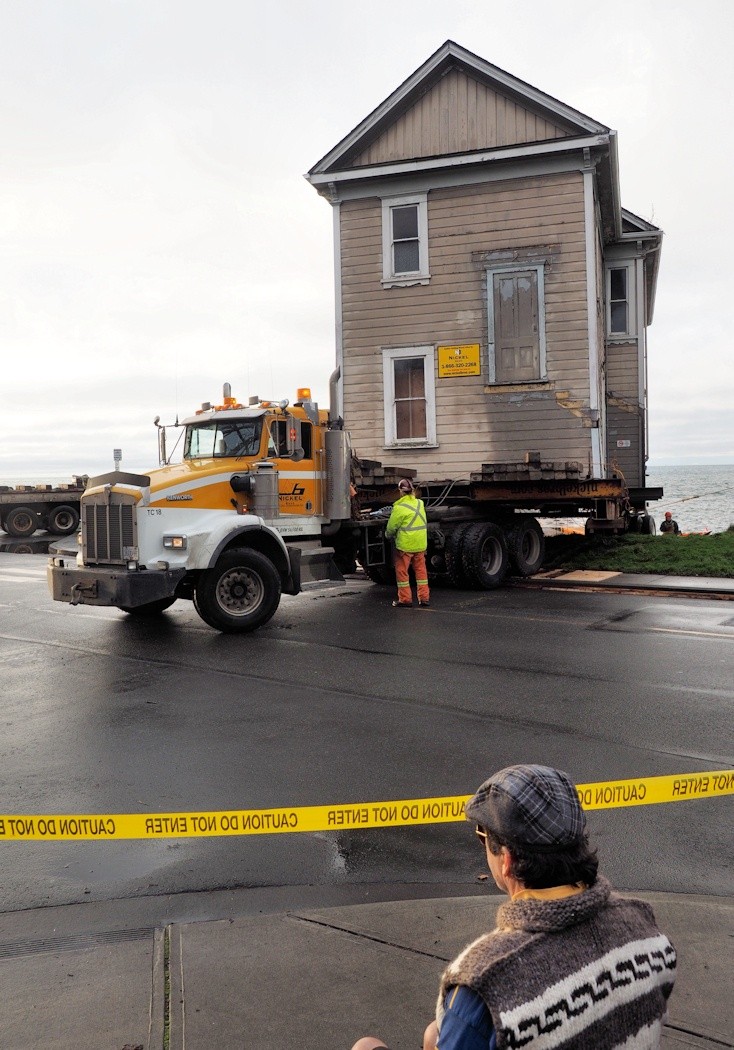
[0,488,86,539]
[43,364,664,637]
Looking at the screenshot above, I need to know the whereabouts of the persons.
[351,764,678,1050]
[659,511,678,536]
[384,478,430,607]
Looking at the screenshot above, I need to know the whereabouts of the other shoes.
[392,600,413,607]
[419,601,431,608]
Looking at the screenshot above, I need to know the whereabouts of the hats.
[465,764,587,848]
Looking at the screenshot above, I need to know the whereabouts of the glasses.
[475,824,489,846]
